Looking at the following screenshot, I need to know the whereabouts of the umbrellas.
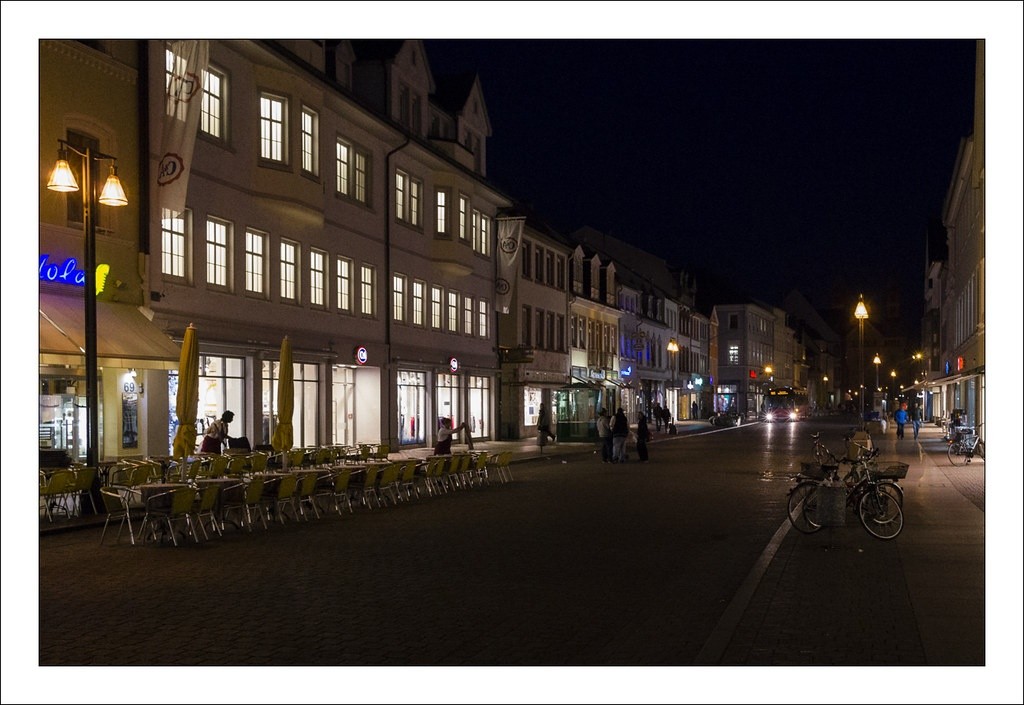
[271,333,294,472]
[168,322,199,462]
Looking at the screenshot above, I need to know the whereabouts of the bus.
[763,387,810,422]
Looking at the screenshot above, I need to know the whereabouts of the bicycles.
[947,422,984,467]
[786,437,910,539]
[807,431,831,466]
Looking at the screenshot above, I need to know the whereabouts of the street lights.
[914,353,925,422]
[823,375,831,412]
[848,293,870,432]
[873,352,881,392]
[46,139,129,515]
[891,369,896,420]
[667,338,679,436]
[900,384,904,405]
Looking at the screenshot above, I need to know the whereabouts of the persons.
[909,402,924,441]
[434,418,464,455]
[595,400,698,464]
[201,411,235,455]
[894,405,908,440]
[535,403,556,442]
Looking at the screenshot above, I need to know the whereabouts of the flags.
[493,219,525,315]
[158,40,209,214]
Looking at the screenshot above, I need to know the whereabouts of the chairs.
[40,443,514,547]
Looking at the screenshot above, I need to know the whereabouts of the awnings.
[901,364,985,393]
[40,289,183,370]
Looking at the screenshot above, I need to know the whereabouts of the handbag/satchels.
[648,432,653,442]
